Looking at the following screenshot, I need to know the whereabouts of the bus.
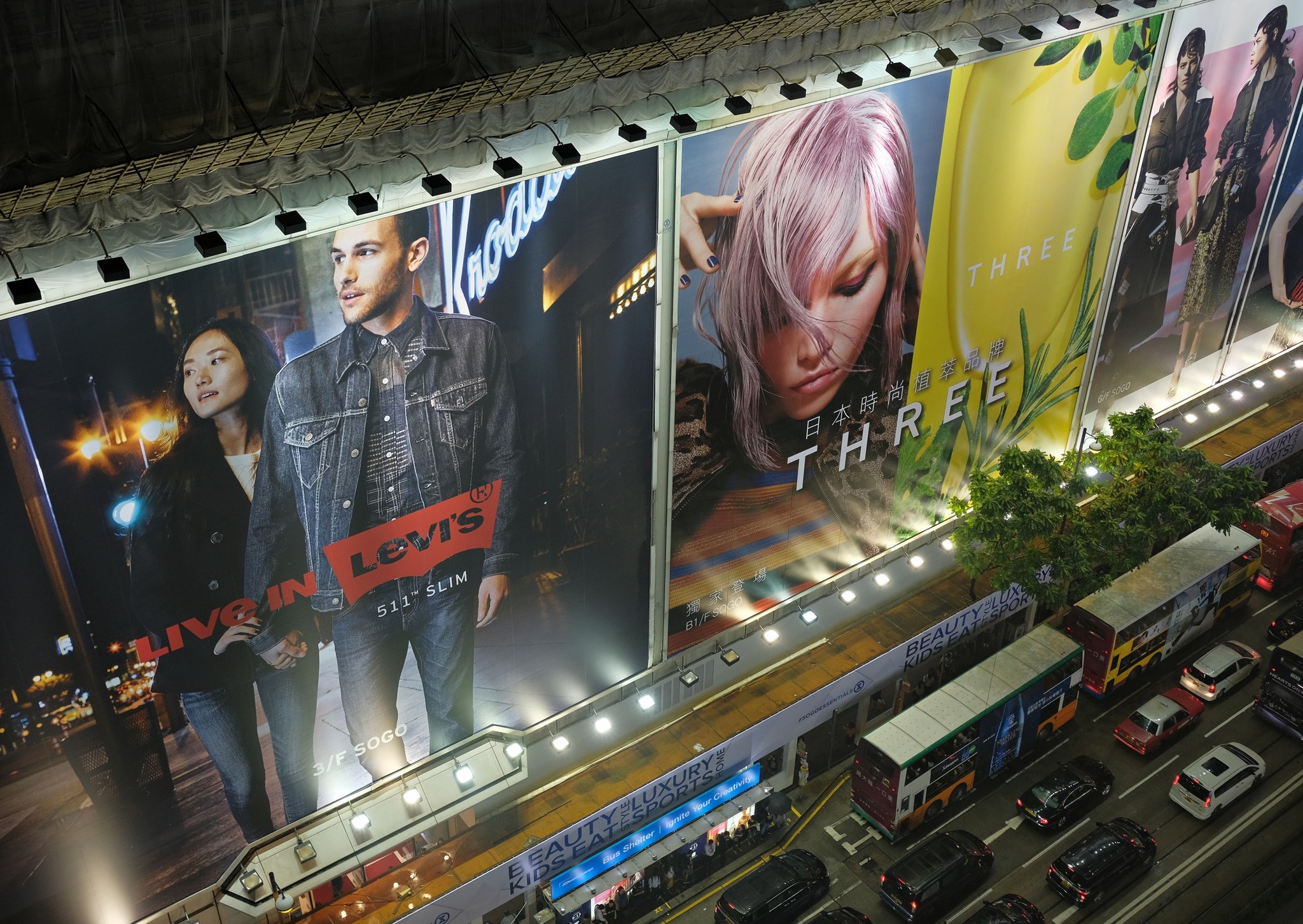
[1238,477,1303,595]
[848,622,1087,845]
[1060,520,1263,703]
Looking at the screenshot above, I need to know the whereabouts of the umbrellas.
[766,793,792,815]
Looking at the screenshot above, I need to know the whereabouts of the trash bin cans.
[56,702,189,864]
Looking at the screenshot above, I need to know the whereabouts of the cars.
[1111,686,1204,756]
[1167,741,1266,821]
[962,893,1045,924]
[1015,754,1114,832]
[1265,601,1303,643]
[1179,640,1263,700]
[804,906,873,924]
[1044,816,1157,909]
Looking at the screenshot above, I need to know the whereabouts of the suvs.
[713,848,830,924]
[879,830,995,924]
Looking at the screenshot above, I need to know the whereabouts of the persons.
[1095,6,1303,449]
[940,652,952,674]
[127,205,517,844]
[1187,576,1225,631]
[316,902,322,907]
[747,813,789,846]
[767,755,777,774]
[436,838,444,846]
[915,671,936,701]
[332,876,343,898]
[1132,631,1149,651]
[421,848,426,853]
[796,736,805,763]
[577,882,644,924]
[844,722,855,747]
[500,909,519,924]
[649,874,660,895]
[679,92,928,474]
[663,861,674,890]
[681,824,746,883]
